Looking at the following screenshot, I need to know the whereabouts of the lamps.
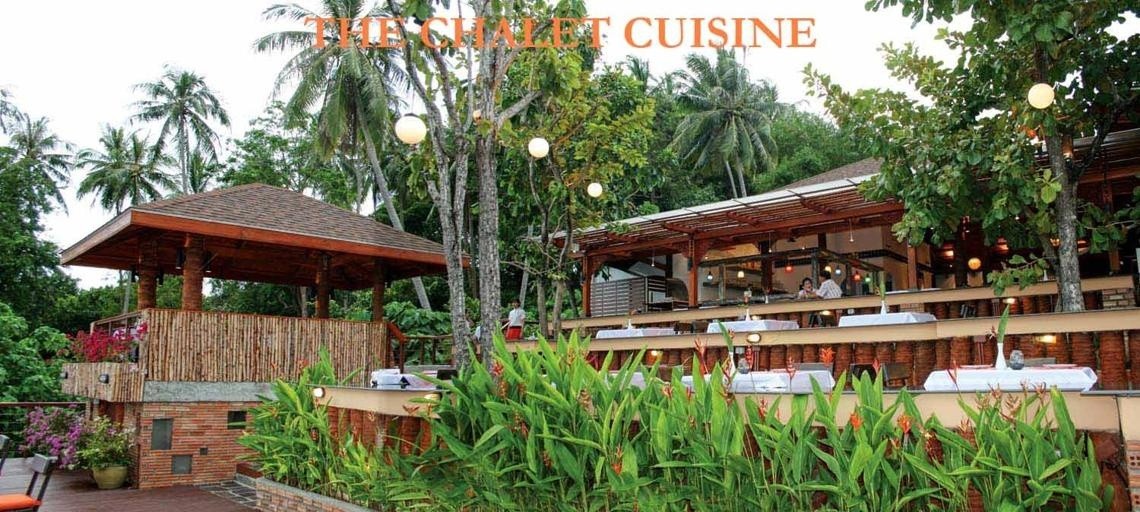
[157,271,164,285]
[130,266,138,283]
[175,247,185,269]
[202,251,211,273]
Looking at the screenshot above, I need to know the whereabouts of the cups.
[1009,349,1025,370]
[737,358,750,374]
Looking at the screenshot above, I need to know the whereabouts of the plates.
[959,364,993,369]
[1043,362,1077,369]
[771,368,796,372]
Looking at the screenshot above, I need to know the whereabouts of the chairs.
[692,321,708,333]
[589,327,613,338]
[817,314,837,328]
[673,322,693,334]
[658,365,674,382]
[796,363,927,394]
[960,304,976,318]
[0,434,57,512]
[808,314,818,327]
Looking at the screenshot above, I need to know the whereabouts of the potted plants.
[878,270,889,315]
[994,303,1011,370]
[76,418,136,490]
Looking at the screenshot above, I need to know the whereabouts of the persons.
[465,309,482,361]
[797,278,820,328]
[503,299,527,340]
[810,271,843,327]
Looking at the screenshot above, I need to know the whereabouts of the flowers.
[56,318,148,364]
[22,403,92,471]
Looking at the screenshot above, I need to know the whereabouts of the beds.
[928,363,1099,393]
